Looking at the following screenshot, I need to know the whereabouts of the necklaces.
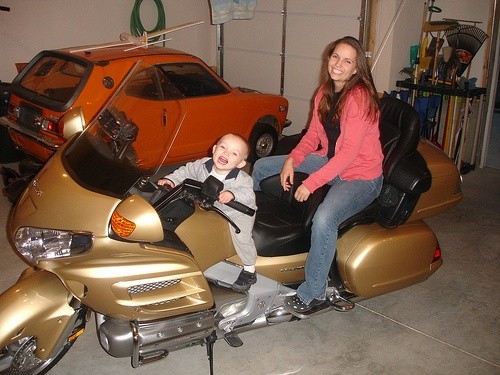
[332,81,345,96]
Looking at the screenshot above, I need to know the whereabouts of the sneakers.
[232,268,257,292]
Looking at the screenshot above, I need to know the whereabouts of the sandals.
[284,294,323,313]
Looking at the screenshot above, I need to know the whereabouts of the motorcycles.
[1,58,465,374]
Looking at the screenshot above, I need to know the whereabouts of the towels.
[209,0,257,25]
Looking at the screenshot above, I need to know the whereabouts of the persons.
[156,132,258,293]
[250,35,384,314]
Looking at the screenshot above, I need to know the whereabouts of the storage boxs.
[337,222,444,300]
[412,138,466,222]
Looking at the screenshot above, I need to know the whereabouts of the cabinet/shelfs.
[395,76,486,175]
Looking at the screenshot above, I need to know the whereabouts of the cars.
[0,42,293,178]
